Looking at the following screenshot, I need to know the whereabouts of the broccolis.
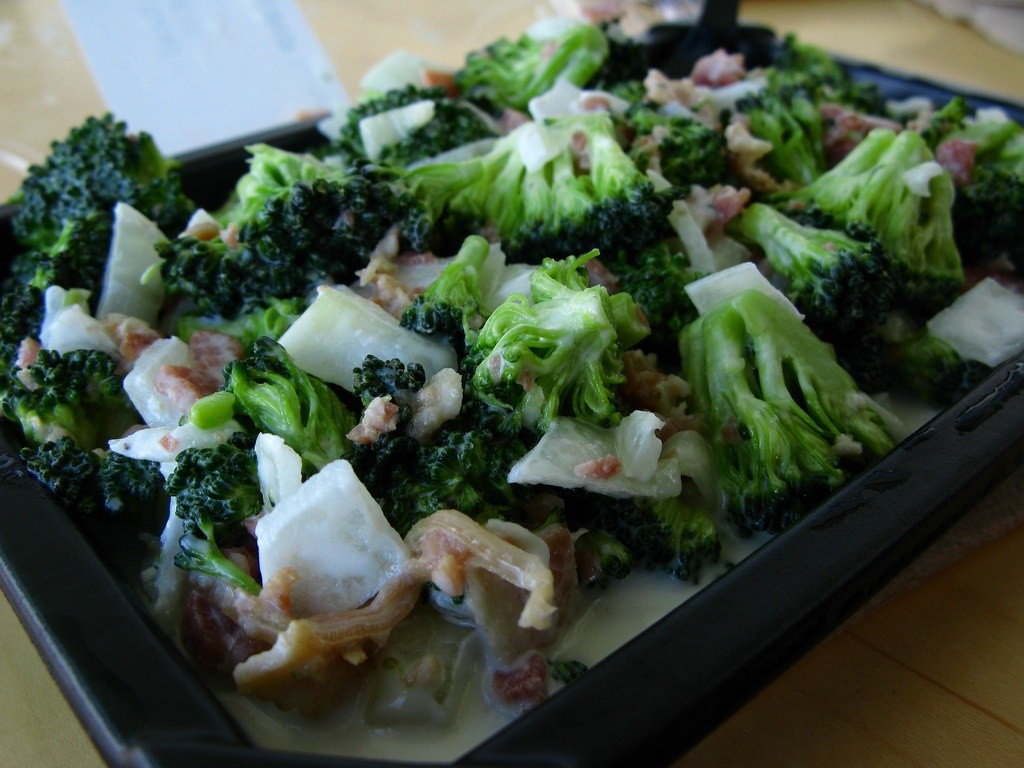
[0,20,1024,594]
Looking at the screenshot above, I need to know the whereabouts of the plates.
[1,32,1024,768]
